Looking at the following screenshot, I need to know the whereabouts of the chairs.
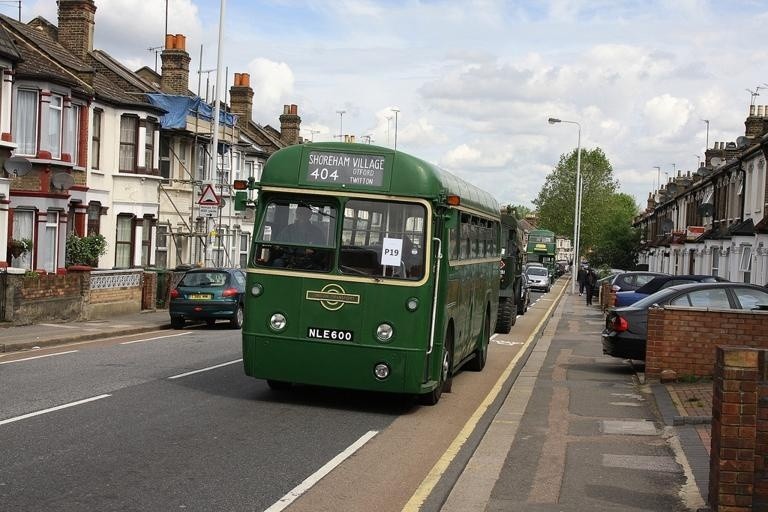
[339,243,415,275]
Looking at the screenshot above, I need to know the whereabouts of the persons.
[266,201,329,270]
[577,264,588,296]
[584,268,597,307]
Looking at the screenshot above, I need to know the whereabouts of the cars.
[579,262,768,362]
[168,266,246,329]
[556,260,569,277]
[525,262,551,293]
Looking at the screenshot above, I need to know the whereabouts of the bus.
[526,227,557,283]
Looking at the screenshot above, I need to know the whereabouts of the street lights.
[547,117,584,296]
